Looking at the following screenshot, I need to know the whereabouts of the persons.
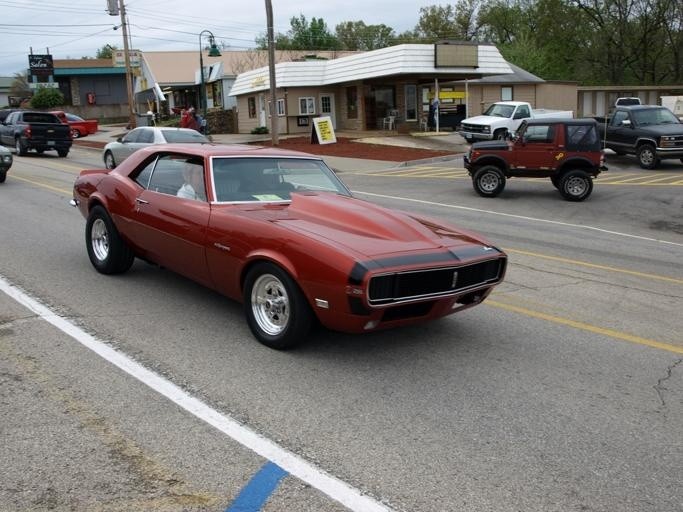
[177,158,207,204]
[178,104,203,134]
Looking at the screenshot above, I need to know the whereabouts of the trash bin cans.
[136,114,152,126]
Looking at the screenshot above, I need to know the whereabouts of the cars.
[0,144,13,183]
[103,127,213,171]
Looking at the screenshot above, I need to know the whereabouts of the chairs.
[257,168,296,194]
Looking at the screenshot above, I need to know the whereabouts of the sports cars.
[70,143,509,350]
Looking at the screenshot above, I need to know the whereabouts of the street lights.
[198,29,222,135]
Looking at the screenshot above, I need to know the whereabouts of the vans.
[614,97,641,110]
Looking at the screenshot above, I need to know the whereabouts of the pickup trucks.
[0,109,98,157]
[458,101,683,202]
